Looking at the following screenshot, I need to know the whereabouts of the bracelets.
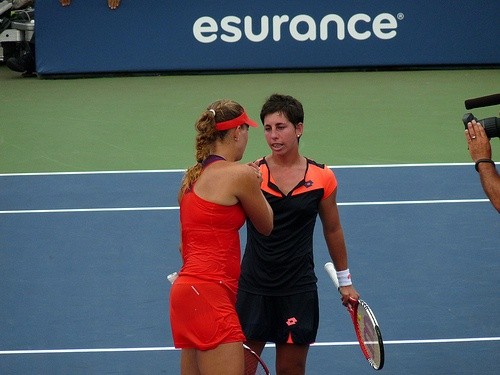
[335,269,352,288]
[474,159,495,171]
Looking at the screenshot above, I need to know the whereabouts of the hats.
[214,112,258,130]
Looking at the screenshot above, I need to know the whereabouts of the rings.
[258,174,262,177]
[472,135,475,138]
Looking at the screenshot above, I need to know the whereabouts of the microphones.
[464,94,500,110]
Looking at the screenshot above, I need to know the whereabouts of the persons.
[234,93,359,375]
[169,100,274,375]
[464,120,500,214]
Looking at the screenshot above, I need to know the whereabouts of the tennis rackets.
[166,273,271,375]
[323,261,385,371]
